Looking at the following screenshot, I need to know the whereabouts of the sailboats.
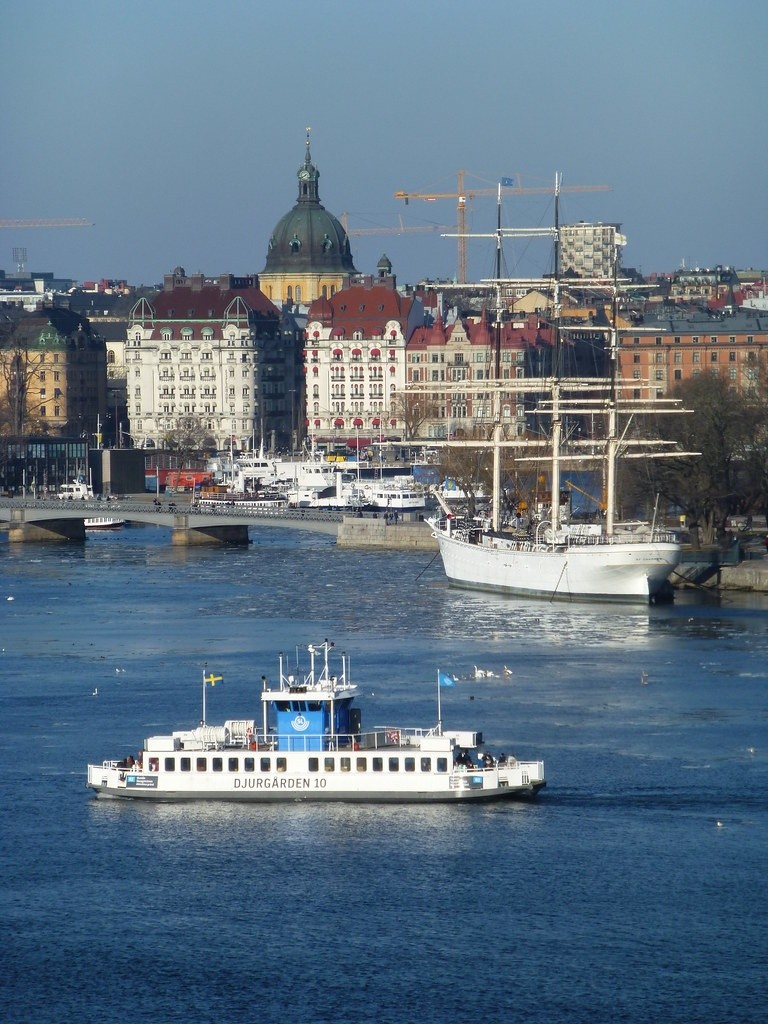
[422,168,681,607]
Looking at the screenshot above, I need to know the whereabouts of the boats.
[84,516,126,529]
[258,415,509,512]
[85,638,546,801]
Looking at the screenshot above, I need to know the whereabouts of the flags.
[439,672,456,687]
[204,670,225,689]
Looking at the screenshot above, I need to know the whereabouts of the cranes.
[1,219,94,227]
[339,170,616,284]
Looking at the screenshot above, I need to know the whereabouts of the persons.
[117,752,142,770]
[152,497,236,515]
[299,503,401,527]
[35,492,113,511]
[454,746,509,771]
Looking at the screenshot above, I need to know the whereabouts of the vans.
[287,449,325,456]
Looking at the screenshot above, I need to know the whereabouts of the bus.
[58,484,94,499]
